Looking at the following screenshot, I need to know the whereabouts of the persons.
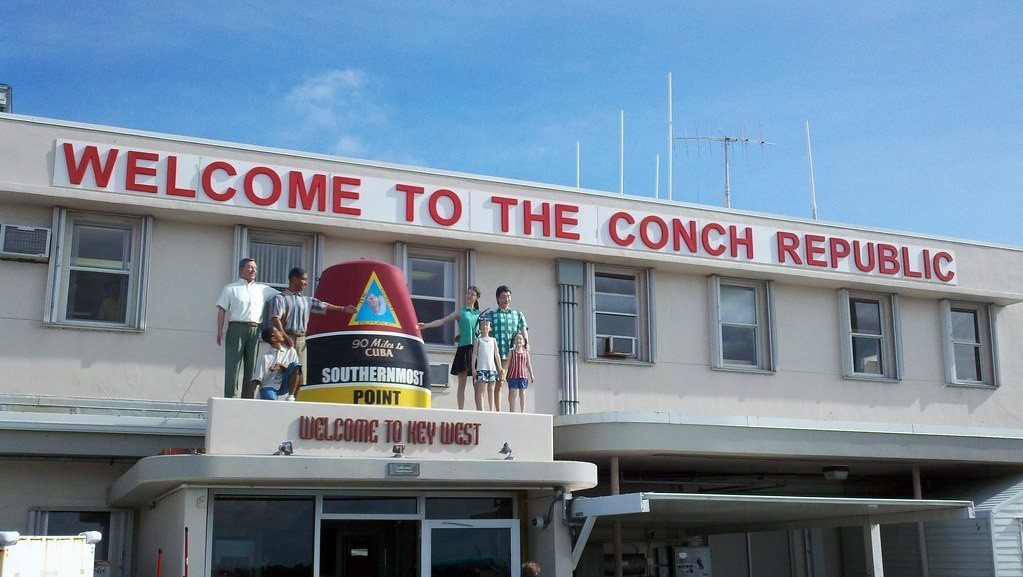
[454,286,530,412]
[216,257,281,399]
[471,317,504,411]
[522,562,540,577]
[97,281,120,321]
[502,332,535,413]
[247,325,302,401]
[418,285,483,410]
[268,267,358,394]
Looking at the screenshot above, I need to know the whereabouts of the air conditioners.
[606,336,636,357]
[0,224,51,264]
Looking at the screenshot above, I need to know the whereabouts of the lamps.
[822,465,850,481]
[392,444,406,458]
[498,442,512,457]
[281,440,293,455]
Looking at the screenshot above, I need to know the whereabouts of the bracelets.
[341,306,343,312]
